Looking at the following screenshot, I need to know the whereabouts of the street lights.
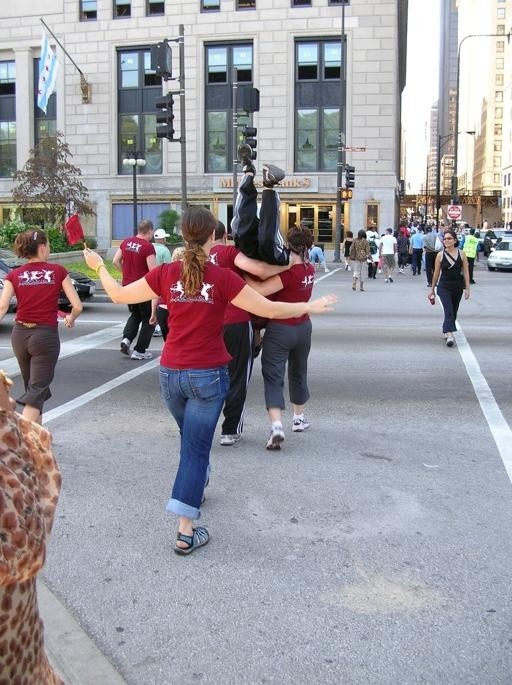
[435,130,476,232]
[453,29,511,206]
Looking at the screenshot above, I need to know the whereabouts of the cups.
[430,295,436,306]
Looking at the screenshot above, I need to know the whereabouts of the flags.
[35,33,61,113]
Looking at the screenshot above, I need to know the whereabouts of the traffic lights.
[242,124,257,161]
[341,189,353,200]
[155,92,172,138]
[348,165,357,191]
[149,41,171,74]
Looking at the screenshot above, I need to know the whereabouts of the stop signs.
[447,205,461,221]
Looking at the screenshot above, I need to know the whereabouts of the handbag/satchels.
[350,243,356,260]
[435,234,443,251]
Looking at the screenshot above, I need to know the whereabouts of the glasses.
[443,237,453,241]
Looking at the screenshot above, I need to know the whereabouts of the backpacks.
[369,239,379,254]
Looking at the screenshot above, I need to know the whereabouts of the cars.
[486,240,511,271]
[1,248,97,313]
[474,228,491,246]
[0,267,18,311]
[445,221,472,235]
[492,235,511,248]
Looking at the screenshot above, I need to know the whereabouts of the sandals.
[175,526,210,555]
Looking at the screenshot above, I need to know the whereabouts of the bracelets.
[96,264,106,274]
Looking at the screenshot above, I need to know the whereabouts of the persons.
[1,231,81,423]
[114,221,186,360]
[430,230,470,345]
[208,143,315,449]
[0,373,73,685]
[342,217,493,290]
[82,206,339,554]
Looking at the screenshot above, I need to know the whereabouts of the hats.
[154,228,170,239]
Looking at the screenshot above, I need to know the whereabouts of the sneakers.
[263,163,279,187]
[237,144,257,176]
[292,419,309,431]
[220,434,241,445]
[120,337,131,355]
[446,336,454,347]
[131,349,152,359]
[389,275,393,282]
[266,428,285,450]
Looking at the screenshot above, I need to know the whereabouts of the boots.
[351,277,357,291]
[360,281,365,291]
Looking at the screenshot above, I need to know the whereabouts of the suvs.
[423,215,436,226]
[484,228,512,256]
